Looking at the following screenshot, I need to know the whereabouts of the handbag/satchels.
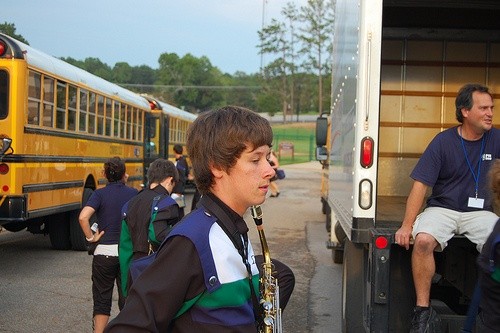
[87,241,98,255]
[276,169,285,179]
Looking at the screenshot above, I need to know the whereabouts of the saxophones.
[250,204,283,333]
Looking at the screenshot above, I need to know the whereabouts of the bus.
[0,32,165,252]
[145,96,198,197]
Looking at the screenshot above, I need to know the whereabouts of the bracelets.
[86,235,94,241]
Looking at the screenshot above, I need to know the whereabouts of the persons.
[266,149,286,198]
[172,144,189,217]
[117,159,181,309]
[78,156,142,333]
[393,83,500,332]
[101,105,276,333]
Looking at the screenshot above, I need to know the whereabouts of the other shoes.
[276,192,280,197]
[270,194,275,196]
[408,303,431,333]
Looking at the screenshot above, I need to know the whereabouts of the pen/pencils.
[483,154,485,160]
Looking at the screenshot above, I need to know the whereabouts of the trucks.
[316,115,333,227]
[316,0,500,333]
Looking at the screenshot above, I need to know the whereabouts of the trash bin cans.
[279,143,294,163]
